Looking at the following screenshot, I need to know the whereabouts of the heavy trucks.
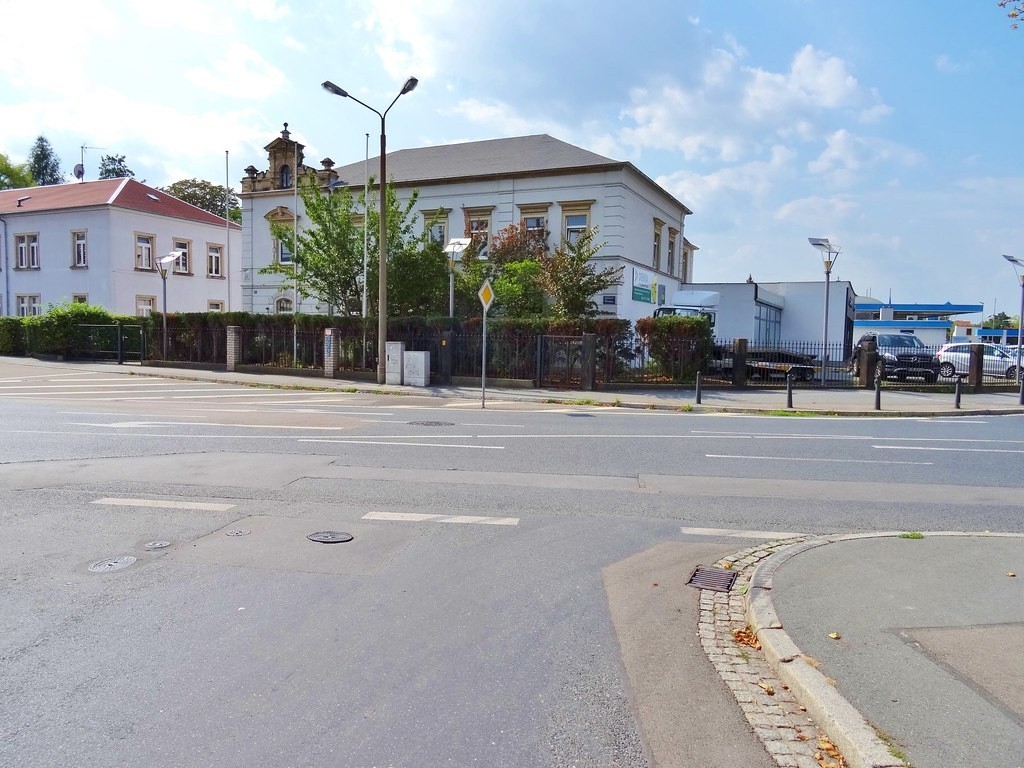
[645,290,817,384]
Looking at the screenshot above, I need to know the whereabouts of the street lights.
[152,247,187,363]
[808,236,843,386]
[320,77,420,386]
[439,236,477,318]
[1001,254,1024,380]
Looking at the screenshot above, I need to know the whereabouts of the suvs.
[851,331,941,383]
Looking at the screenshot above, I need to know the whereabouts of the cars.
[934,342,1024,381]
